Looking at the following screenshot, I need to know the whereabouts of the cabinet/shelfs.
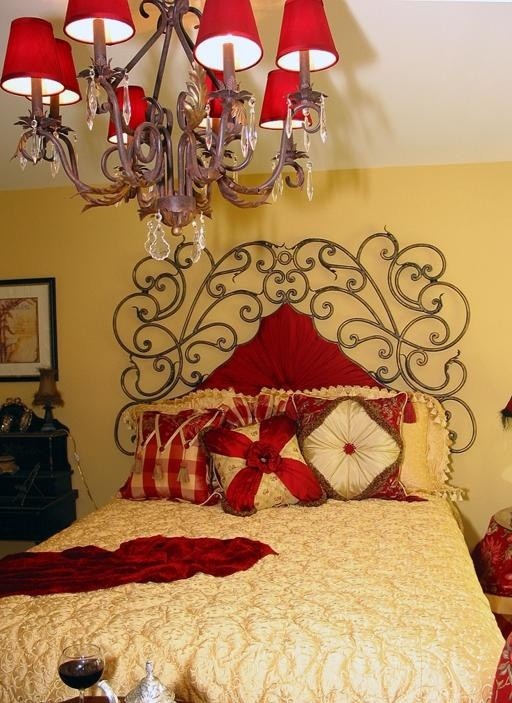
[0,398,79,547]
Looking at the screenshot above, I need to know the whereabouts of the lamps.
[0,0,340,264]
[31,368,65,432]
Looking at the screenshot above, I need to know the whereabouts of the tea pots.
[99,661,178,703]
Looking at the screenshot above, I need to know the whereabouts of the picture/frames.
[0,277,59,381]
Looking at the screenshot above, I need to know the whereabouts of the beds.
[0,226,506,703]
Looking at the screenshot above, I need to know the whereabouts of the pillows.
[116,409,226,506]
[256,384,465,502]
[122,388,255,439]
[291,392,428,502]
[199,415,327,516]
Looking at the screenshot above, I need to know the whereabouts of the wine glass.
[56,643,106,703]
